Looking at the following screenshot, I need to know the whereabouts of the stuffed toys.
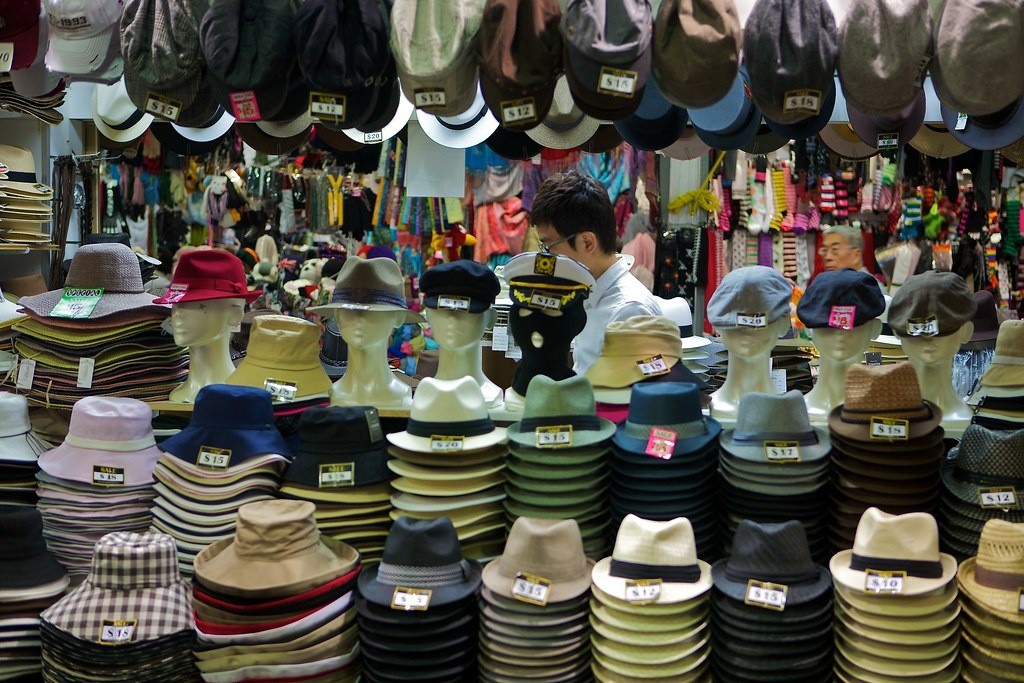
[252,237,345,309]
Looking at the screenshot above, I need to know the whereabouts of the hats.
[0,385,1024,683]
[0,0,1024,161]
[419,260,501,313]
[653,295,711,383]
[707,266,794,331]
[963,319,1024,430]
[305,255,429,325]
[771,327,813,393]
[152,250,263,304]
[1,144,191,411]
[967,289,1000,342]
[796,267,886,329]
[888,270,976,337]
[584,314,713,423]
[504,252,597,309]
[225,315,333,416]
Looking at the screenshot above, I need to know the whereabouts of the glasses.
[817,246,857,256]
[539,234,578,252]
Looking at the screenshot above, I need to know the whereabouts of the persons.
[796,267,886,421]
[888,270,977,421]
[418,260,503,409]
[707,265,794,419]
[530,170,663,378]
[503,252,596,412]
[152,249,263,405]
[305,256,412,409]
[818,225,890,296]
